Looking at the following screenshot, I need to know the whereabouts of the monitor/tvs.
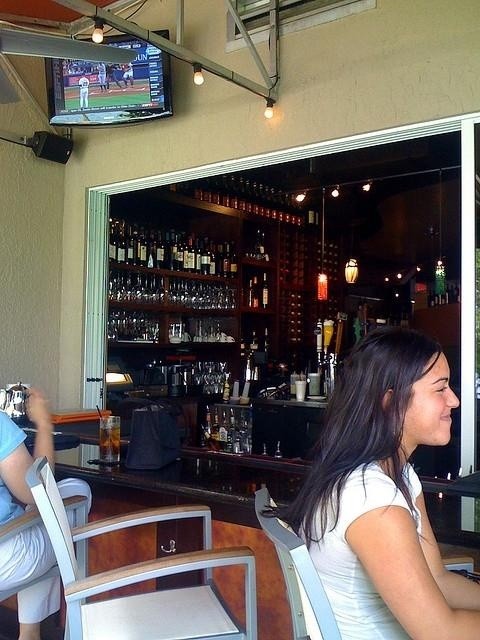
[44,28,174,127]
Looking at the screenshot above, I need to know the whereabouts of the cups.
[295,374,321,401]
[99,416,121,464]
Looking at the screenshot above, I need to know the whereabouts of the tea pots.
[0,377,29,425]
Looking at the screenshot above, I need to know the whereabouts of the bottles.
[428,283,460,306]
[109,217,237,278]
[201,403,253,453]
[194,174,304,226]
[290,370,307,394]
[241,229,269,382]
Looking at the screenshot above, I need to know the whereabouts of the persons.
[259,325,480,640]
[106,64,122,89]
[0,387,75,640]
[78,73,90,110]
[97,62,106,90]
[121,63,134,88]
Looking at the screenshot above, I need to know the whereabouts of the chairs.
[254,488,475,640]
[0,495,87,640]
[25,457,258,640]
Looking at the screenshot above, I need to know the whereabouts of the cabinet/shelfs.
[109,174,341,438]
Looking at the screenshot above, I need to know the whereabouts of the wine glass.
[192,361,231,394]
[106,270,239,343]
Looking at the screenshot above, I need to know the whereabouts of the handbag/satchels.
[126,404,181,469]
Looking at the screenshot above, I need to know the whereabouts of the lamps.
[193,62,205,85]
[344,184,358,285]
[265,97,276,117]
[317,187,328,301]
[433,170,446,295]
[91,19,106,43]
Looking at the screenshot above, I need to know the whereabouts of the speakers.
[32,130,73,165]
